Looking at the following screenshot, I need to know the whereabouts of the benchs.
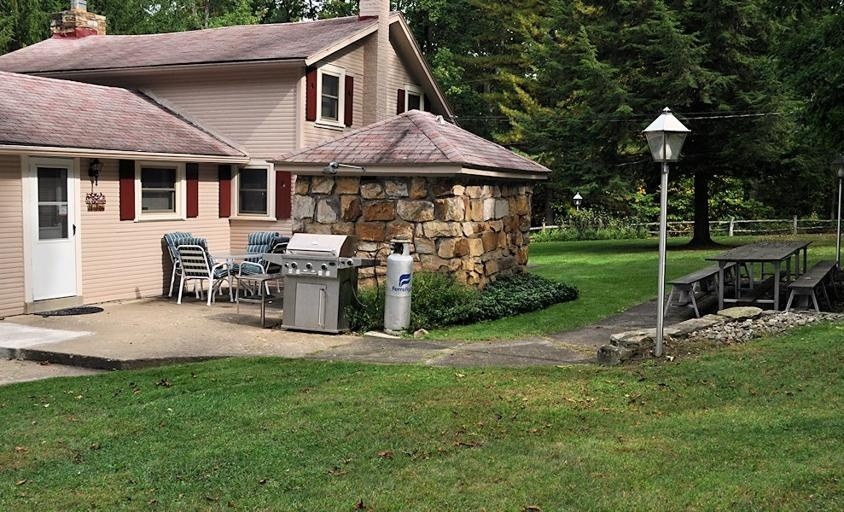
[784,260,840,312]
[663,262,751,317]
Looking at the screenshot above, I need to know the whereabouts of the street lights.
[572,192,584,213]
[829,151,843,271]
[642,107,692,356]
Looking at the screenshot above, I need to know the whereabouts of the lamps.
[89,158,103,185]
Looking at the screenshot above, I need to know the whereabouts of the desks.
[705,241,812,311]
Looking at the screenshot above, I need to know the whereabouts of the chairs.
[164,232,290,306]
